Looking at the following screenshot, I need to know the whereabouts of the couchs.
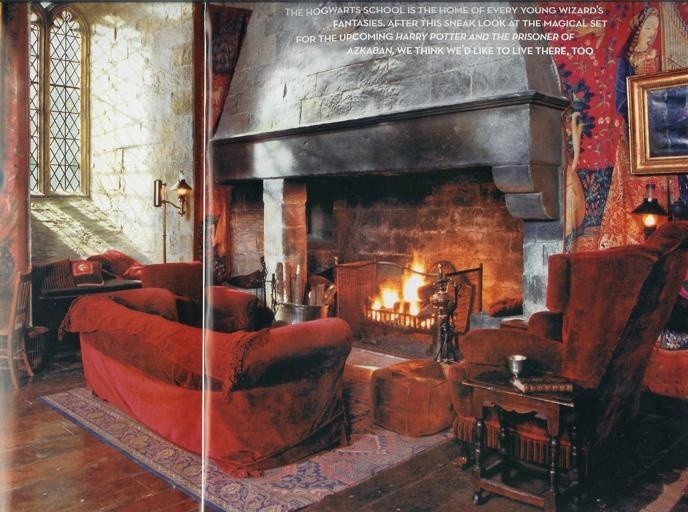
[58,287,354,477]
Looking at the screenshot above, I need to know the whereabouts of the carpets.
[39,347,452,512]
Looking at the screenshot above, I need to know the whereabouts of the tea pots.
[428,275,461,321]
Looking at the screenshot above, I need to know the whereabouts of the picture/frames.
[626,67,688,176]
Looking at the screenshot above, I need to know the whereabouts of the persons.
[611,5,662,123]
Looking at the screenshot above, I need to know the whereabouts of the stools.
[369,359,456,437]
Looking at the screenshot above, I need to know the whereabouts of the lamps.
[153,170,194,217]
[633,182,667,232]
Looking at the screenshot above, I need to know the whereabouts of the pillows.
[33,249,145,296]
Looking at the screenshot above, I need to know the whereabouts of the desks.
[462,370,598,512]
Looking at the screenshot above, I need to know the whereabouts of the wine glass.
[507,354,528,385]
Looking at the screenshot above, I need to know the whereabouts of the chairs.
[141,261,258,332]
[448,219,687,474]
[0,267,35,390]
